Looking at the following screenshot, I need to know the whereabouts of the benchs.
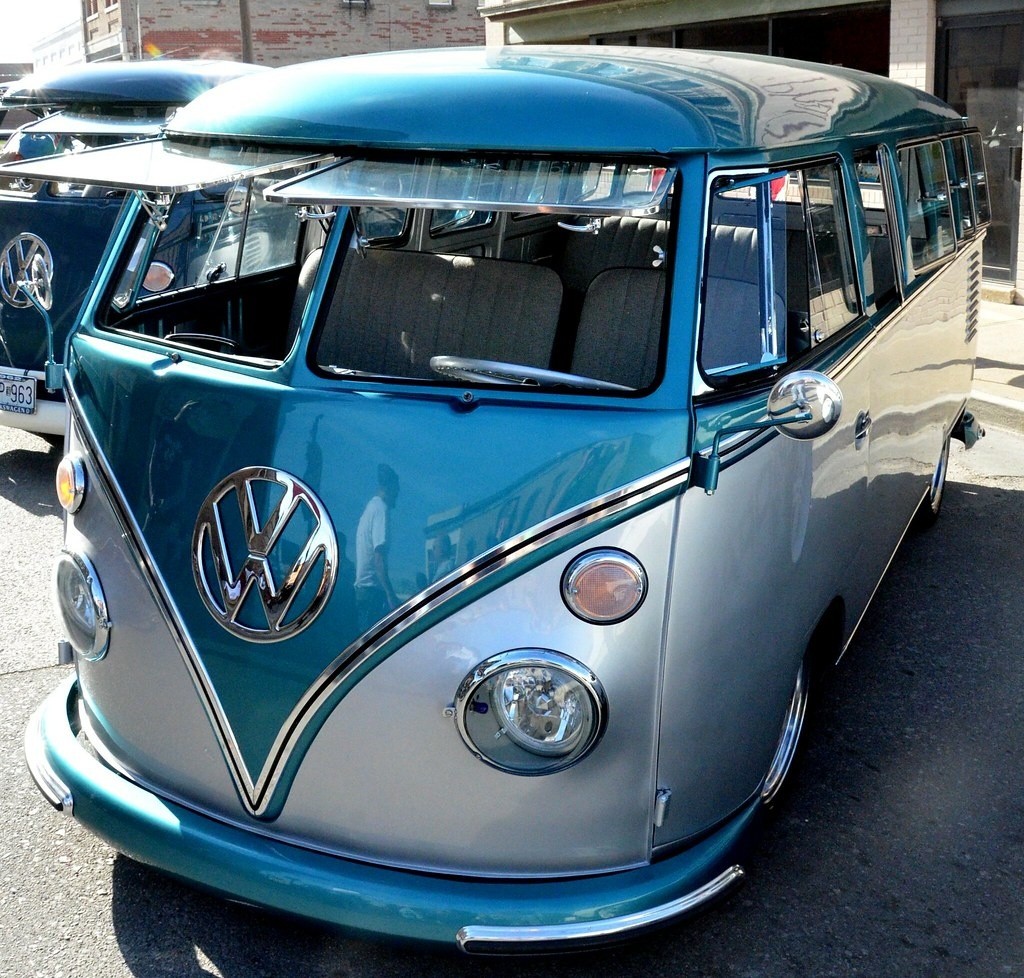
[285,213,931,392]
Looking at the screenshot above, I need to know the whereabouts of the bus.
[14,42,992,957]
[0,59,276,451]
